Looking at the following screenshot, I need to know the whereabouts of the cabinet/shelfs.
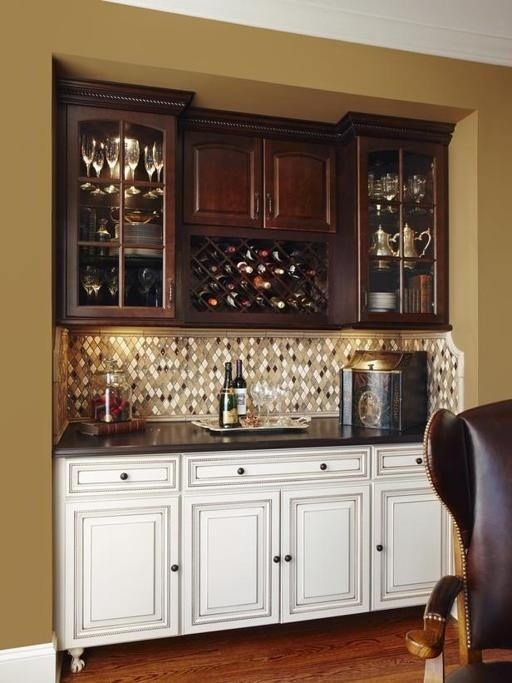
[330,111,456,331]
[378,444,462,610]
[57,451,185,650]
[55,78,190,327]
[180,107,342,331]
[185,447,376,638]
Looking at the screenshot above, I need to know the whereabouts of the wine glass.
[368,171,430,217]
[90,140,106,196]
[80,263,163,307]
[151,140,167,195]
[104,135,121,194]
[80,133,96,192]
[124,137,142,194]
[142,142,158,199]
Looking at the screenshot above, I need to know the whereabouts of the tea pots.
[393,220,431,271]
[369,224,400,272]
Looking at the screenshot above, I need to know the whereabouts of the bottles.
[231,358,248,417]
[196,240,319,311]
[90,355,134,422]
[218,360,239,429]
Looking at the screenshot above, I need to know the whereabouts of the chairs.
[403,396,511,682]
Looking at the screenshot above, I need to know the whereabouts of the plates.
[367,290,396,313]
[110,220,163,258]
